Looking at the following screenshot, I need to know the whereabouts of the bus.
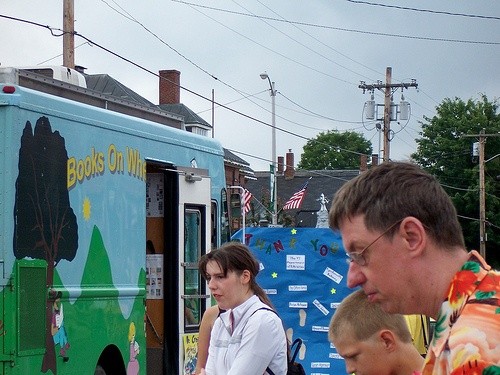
[1,82,230,374]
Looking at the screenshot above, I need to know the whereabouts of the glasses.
[345,218,432,268]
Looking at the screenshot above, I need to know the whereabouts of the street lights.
[463,129,496,262]
[361,69,417,161]
[260,73,280,226]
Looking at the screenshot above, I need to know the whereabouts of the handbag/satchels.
[241,308,307,375]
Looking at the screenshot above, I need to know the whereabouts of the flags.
[239,183,252,221]
[282,178,310,210]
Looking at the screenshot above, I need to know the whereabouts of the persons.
[193,241,291,375]
[327,288,425,375]
[328,158,500,375]
